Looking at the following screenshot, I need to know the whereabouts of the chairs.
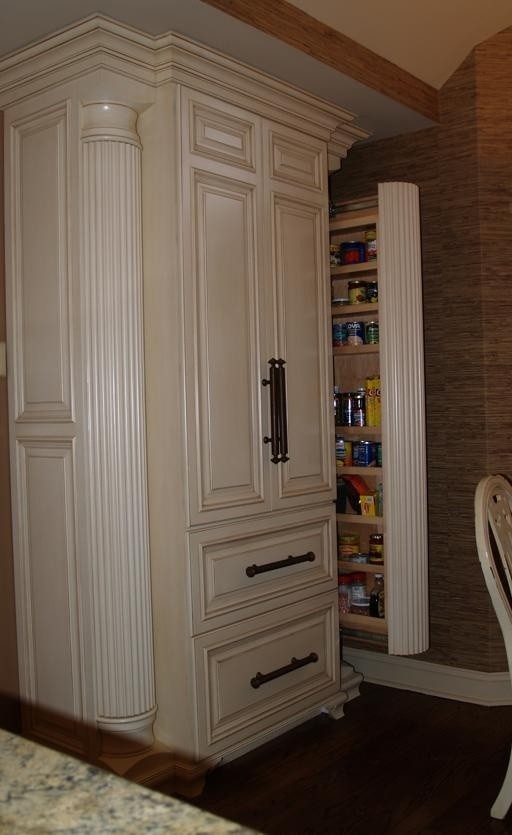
[471,473,512,821]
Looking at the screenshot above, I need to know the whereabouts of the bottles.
[333,389,367,428]
[338,570,384,619]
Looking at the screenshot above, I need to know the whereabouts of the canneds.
[333,280,379,346]
[336,437,382,466]
[333,392,365,426]
[369,534,384,564]
[330,230,377,268]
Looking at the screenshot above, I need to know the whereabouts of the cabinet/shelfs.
[153,499,348,798]
[328,182,433,657]
[135,82,339,531]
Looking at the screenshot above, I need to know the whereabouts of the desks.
[1,730,264,834]
